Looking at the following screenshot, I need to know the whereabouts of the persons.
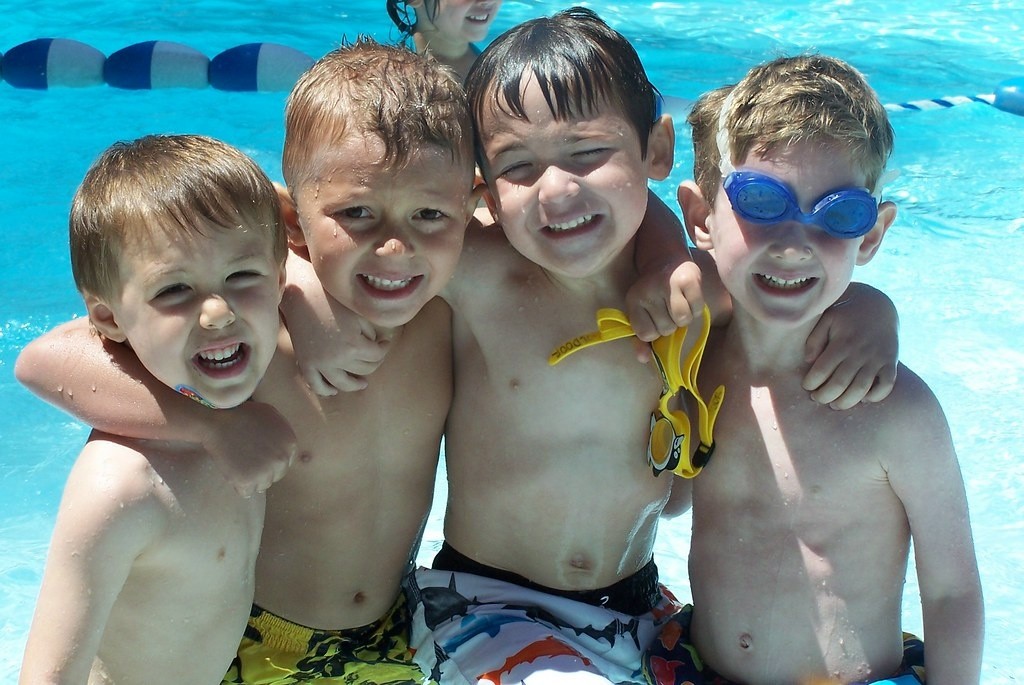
[15,31,705,685]
[16,134,290,685]
[640,54,985,685]
[278,7,899,685]
[384,0,502,85]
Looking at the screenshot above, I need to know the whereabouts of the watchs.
[549,302,725,480]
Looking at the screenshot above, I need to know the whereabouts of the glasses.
[716,73,902,239]
[550,302,725,478]
